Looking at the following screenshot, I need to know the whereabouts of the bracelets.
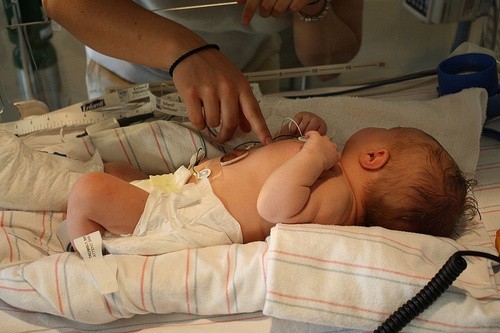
[297,0,330,23]
[168,44,220,77]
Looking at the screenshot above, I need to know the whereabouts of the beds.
[0,61,500,333]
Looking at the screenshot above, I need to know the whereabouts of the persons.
[41,0,365,147]
[67,111,481,259]
